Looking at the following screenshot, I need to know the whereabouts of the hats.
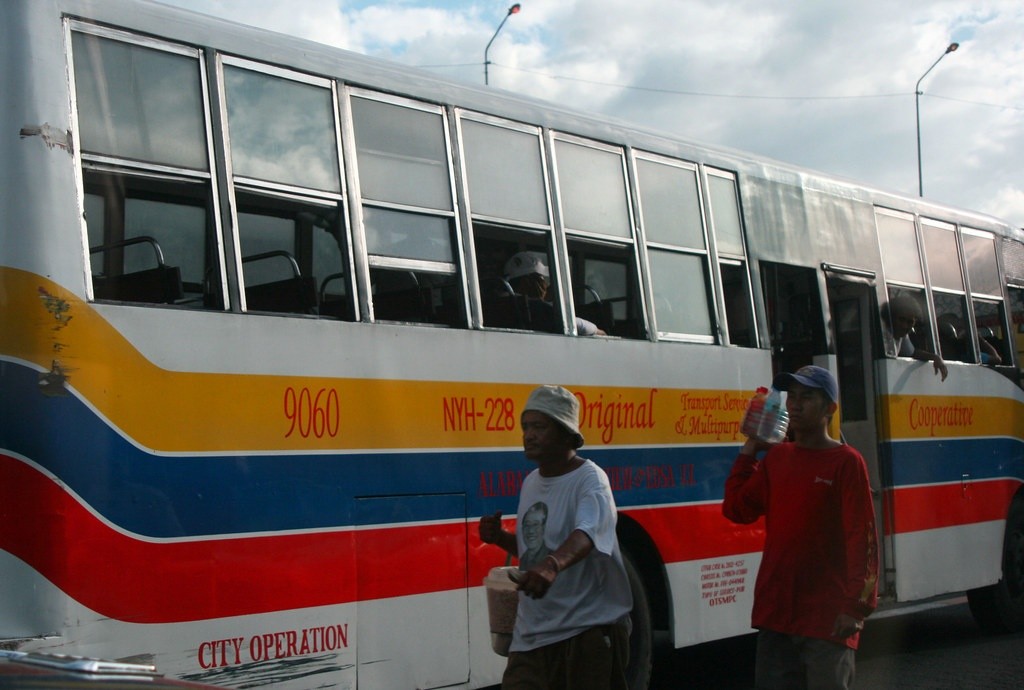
[783,365,837,406]
[518,386,585,449]
[503,252,549,281]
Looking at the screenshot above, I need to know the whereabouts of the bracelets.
[546,555,560,571]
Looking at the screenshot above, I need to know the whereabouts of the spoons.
[508,568,524,584]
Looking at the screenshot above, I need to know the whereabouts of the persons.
[479,386,633,690]
[937,317,1002,364]
[519,501,554,571]
[889,295,948,381]
[503,252,607,336]
[722,365,879,690]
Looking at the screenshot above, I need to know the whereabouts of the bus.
[0,0,1024,690]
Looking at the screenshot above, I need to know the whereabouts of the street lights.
[484,3,522,87]
[915,42,960,198]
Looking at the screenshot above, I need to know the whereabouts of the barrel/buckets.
[483,535,520,656]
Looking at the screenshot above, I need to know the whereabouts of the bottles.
[757,385,781,440]
[764,390,789,442]
[740,386,768,437]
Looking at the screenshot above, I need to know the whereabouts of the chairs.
[580,285,611,335]
[202,251,320,316]
[319,270,421,322]
[603,295,646,341]
[421,276,530,329]
[90,236,192,307]
